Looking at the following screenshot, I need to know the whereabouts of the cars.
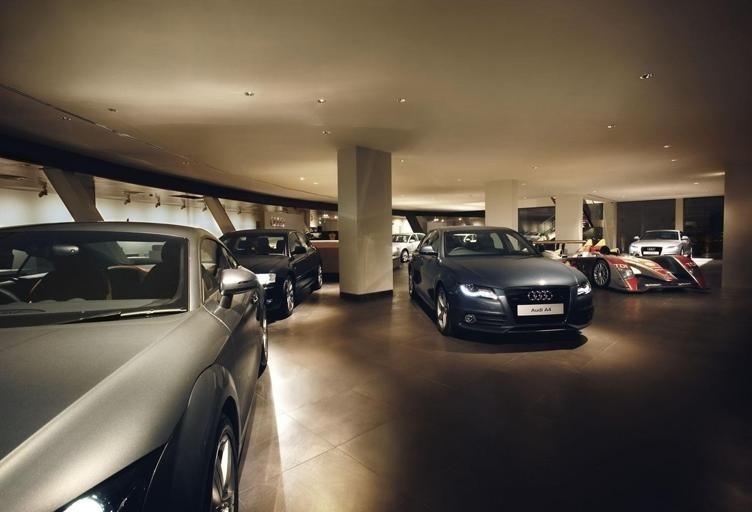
[629,229,694,258]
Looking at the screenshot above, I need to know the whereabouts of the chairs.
[396,237,404,242]
[30,239,181,301]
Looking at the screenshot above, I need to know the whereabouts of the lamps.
[180,199,186,210]
[202,204,208,212]
[124,192,133,205]
[155,195,162,208]
[236,207,242,215]
[38,181,49,198]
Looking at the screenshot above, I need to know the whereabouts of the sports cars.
[544,239,706,294]
